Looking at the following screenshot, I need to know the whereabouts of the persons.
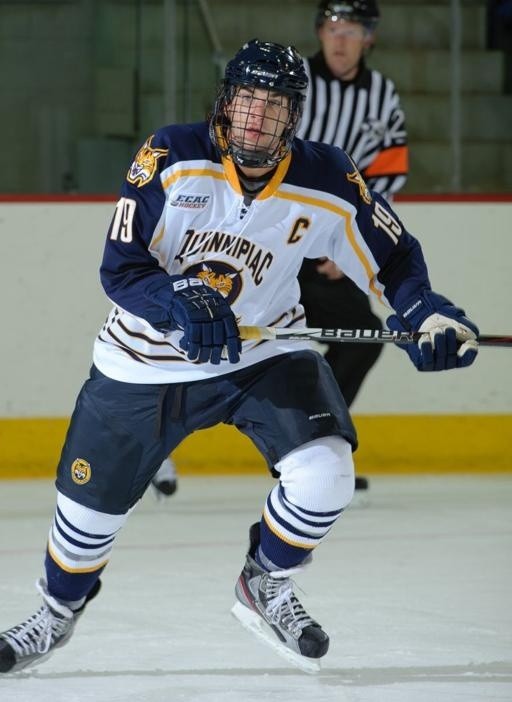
[0,40,479,674]
[151,0,409,496]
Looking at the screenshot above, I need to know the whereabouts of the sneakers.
[153,457,177,496]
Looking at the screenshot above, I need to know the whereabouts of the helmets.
[315,1,381,39]
[208,37,310,168]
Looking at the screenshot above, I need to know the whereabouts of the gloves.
[144,272,242,365]
[387,292,481,371]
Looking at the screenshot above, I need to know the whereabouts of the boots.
[1,577,101,672]
[234,522,330,659]
